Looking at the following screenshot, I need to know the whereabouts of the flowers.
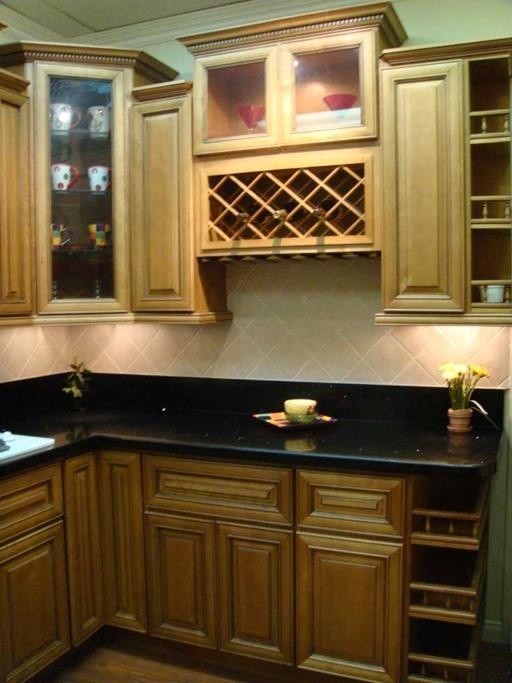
[439,358,488,411]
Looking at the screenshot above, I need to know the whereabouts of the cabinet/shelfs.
[194,19,405,263]
[35,52,124,313]
[1,435,104,683]
[128,82,234,312]
[104,442,294,666]
[293,470,496,681]
[378,42,510,316]
[2,87,37,324]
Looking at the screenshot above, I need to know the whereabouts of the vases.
[444,408,474,432]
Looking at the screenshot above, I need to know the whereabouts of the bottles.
[487,283,505,303]
[273,202,301,222]
[236,211,263,223]
[313,201,339,220]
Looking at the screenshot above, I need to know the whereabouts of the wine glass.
[235,104,265,134]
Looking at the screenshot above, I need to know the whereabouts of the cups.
[86,166,112,192]
[51,162,80,191]
[51,103,83,135]
[87,104,111,142]
[51,221,73,253]
[89,224,112,251]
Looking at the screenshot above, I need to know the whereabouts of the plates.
[253,411,339,427]
[286,415,316,423]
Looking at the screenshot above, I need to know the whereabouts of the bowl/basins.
[285,435,320,454]
[284,399,317,414]
[320,93,357,110]
